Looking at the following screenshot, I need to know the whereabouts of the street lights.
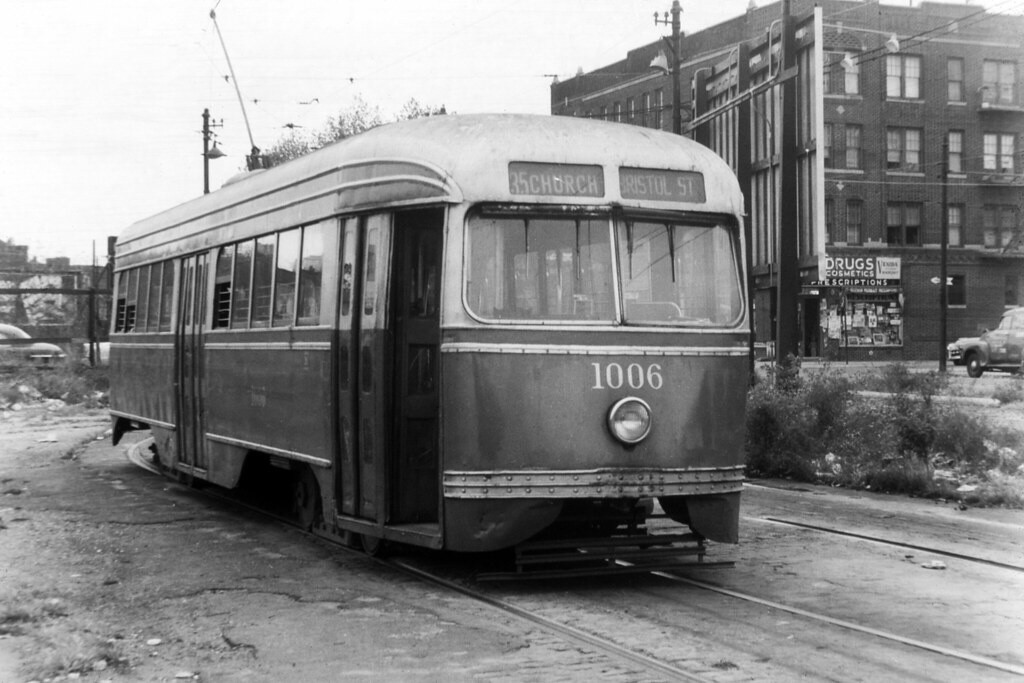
[201,131,227,195]
[650,34,684,134]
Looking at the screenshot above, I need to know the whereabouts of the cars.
[0,324,67,372]
[960,305,1023,379]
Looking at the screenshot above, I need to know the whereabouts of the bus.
[106,113,756,579]
